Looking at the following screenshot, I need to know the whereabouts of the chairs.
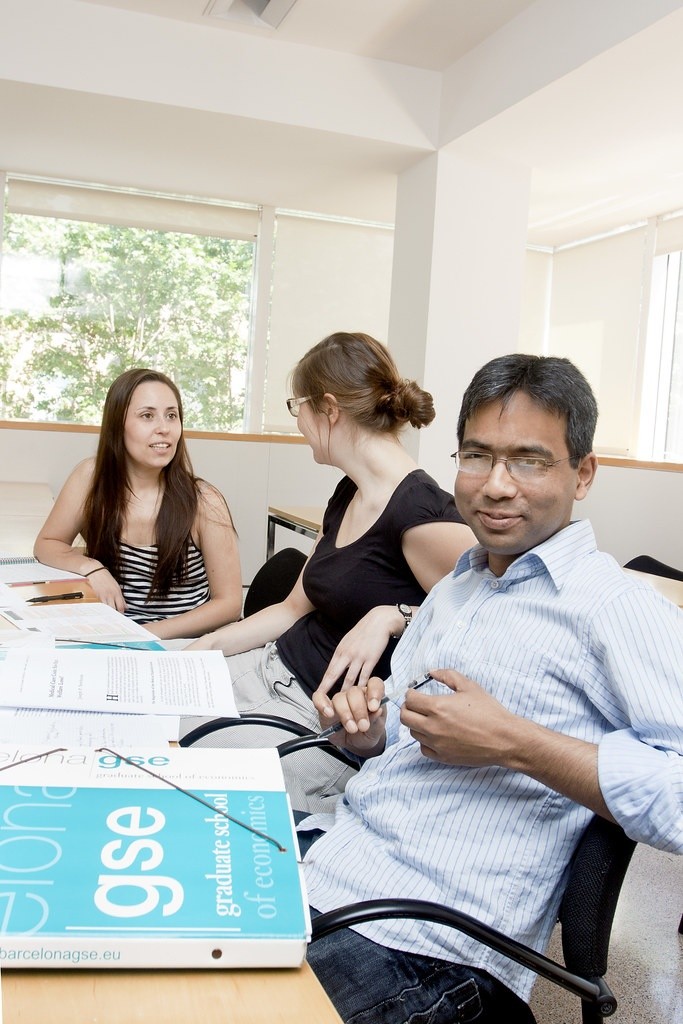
[241,547,308,621]
[277,555,682,1023]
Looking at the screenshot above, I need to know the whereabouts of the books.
[0,743,314,973]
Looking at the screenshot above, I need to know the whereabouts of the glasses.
[451,450,579,483]
[287,393,323,417]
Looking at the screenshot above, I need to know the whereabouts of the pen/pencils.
[26,592,85,603]
[317,673,433,739]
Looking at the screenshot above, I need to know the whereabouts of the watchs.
[392,601,415,642]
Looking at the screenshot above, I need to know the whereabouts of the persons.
[157,332,480,815]
[304,354,682,1024]
[33,368,244,638]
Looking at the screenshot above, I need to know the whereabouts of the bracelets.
[85,566,109,577]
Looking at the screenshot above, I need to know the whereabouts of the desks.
[0,480,344,1024]
[264,503,323,561]
